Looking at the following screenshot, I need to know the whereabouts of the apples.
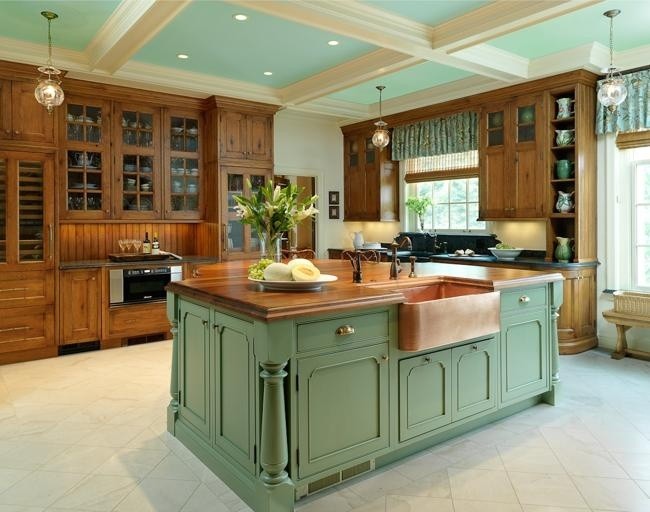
[495,244,511,249]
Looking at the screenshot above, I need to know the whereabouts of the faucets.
[424,232,448,253]
[390,236,413,278]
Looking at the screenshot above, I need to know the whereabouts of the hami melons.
[263,258,320,282]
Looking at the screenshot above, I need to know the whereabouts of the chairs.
[340,249,378,262]
[282,249,316,259]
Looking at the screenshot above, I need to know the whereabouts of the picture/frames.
[328,190,340,205]
[329,205,339,219]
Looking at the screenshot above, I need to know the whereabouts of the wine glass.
[119,239,141,255]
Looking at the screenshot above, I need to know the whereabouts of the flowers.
[230,176,306,234]
[258,178,321,234]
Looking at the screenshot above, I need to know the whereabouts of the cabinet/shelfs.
[292,306,390,481]
[327,248,387,262]
[57,267,102,343]
[111,81,206,226]
[177,297,258,477]
[339,117,402,223]
[547,67,599,264]
[502,283,552,406]
[476,75,546,221]
[0,271,59,366]
[196,160,272,261]
[399,335,502,442]
[0,147,56,272]
[0,58,69,152]
[203,93,277,164]
[430,259,597,356]
[55,75,110,225]
[101,301,173,339]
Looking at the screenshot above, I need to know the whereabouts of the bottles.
[493,112,503,126]
[142,232,150,254]
[152,232,160,255]
[520,108,534,123]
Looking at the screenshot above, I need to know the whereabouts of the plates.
[248,272,338,292]
[66,113,198,212]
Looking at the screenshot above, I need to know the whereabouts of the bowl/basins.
[364,242,381,248]
[488,247,524,261]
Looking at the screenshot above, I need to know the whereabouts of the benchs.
[601,309,650,362]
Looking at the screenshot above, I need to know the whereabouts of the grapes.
[247,258,273,280]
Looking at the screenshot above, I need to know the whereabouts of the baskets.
[612,290,650,318]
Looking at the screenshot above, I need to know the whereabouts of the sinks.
[391,249,434,262]
[359,276,501,353]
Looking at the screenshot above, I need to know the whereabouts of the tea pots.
[349,231,365,248]
[555,98,575,263]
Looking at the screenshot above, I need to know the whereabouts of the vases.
[271,233,283,263]
[256,232,271,260]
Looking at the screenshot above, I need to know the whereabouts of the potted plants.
[404,196,434,234]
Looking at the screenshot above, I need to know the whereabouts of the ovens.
[109,267,182,306]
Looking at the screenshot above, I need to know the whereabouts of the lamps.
[369,84,392,154]
[31,9,68,116]
[595,8,629,116]
